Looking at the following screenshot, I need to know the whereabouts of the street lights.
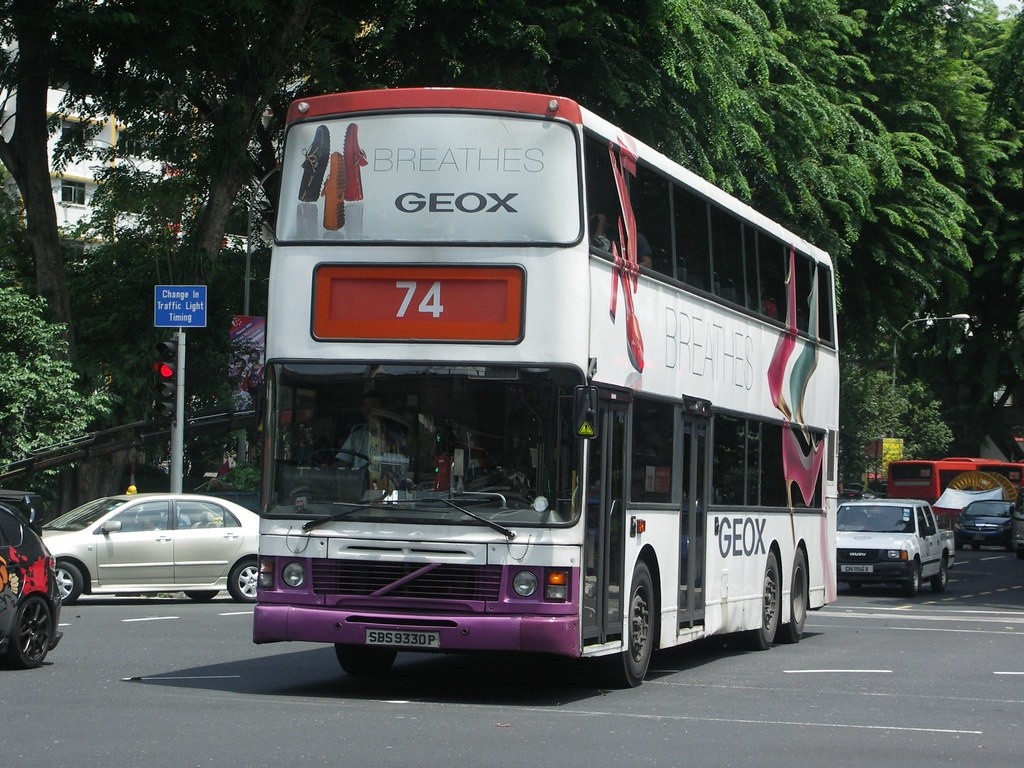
[889,312,970,436]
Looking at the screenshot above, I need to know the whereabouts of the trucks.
[1009,485,1024,559]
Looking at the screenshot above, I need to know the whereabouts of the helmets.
[126,486,137,494]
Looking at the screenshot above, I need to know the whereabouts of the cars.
[0,488,64,670]
[842,480,887,498]
[952,499,1014,552]
[40,485,259,606]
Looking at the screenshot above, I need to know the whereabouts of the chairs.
[651,246,808,332]
[177,512,218,528]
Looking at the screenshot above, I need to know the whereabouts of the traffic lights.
[157,338,175,422]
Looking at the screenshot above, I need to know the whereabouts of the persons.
[337,398,416,492]
[126,486,161,532]
[625,232,656,266]
[228,350,264,411]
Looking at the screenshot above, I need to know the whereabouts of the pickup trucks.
[837,498,957,598]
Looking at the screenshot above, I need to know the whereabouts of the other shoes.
[299,124,329,202]
[321,153,345,230]
[343,123,368,201]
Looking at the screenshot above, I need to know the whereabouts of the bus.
[886,457,1024,507]
[255,87,840,690]
